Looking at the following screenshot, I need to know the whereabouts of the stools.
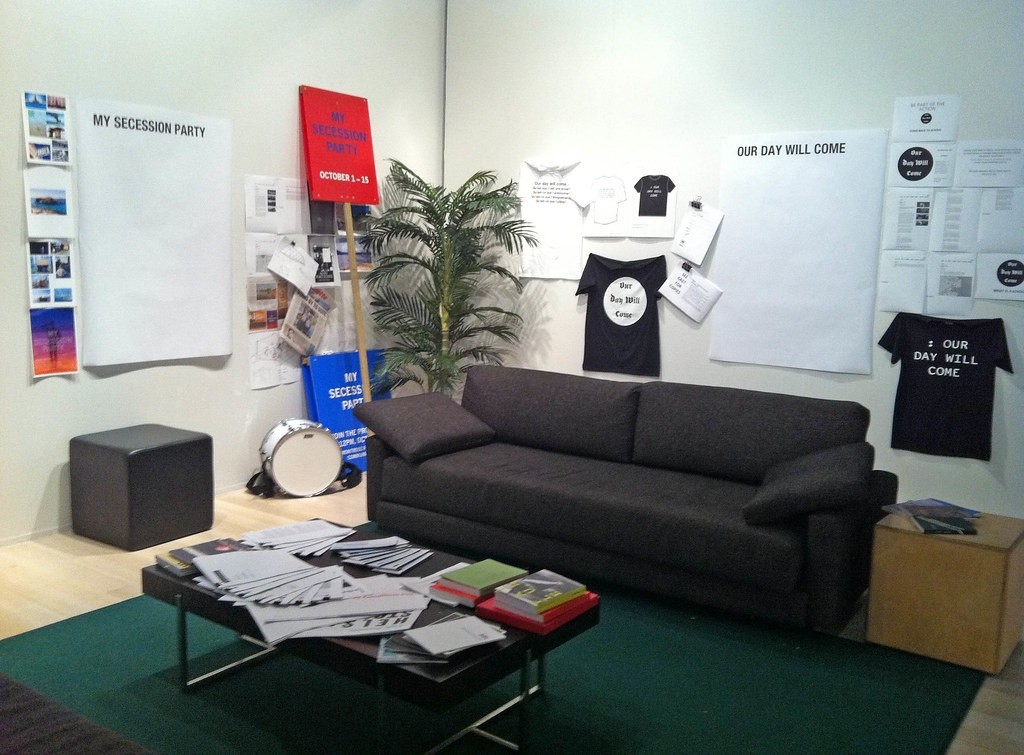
[68,423,215,552]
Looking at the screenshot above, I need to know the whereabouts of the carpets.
[0,520,993,755]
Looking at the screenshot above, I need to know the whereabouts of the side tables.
[866,500,1024,675]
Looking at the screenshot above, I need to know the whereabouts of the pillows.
[351,389,498,466]
[740,443,876,527]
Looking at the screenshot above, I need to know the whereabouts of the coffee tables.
[139,516,601,755]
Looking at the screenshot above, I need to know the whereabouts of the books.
[881,497,983,520]
[898,503,979,535]
[155,516,598,665]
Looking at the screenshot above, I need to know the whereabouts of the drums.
[259,418,340,497]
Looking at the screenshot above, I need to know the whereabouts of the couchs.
[364,362,901,641]
